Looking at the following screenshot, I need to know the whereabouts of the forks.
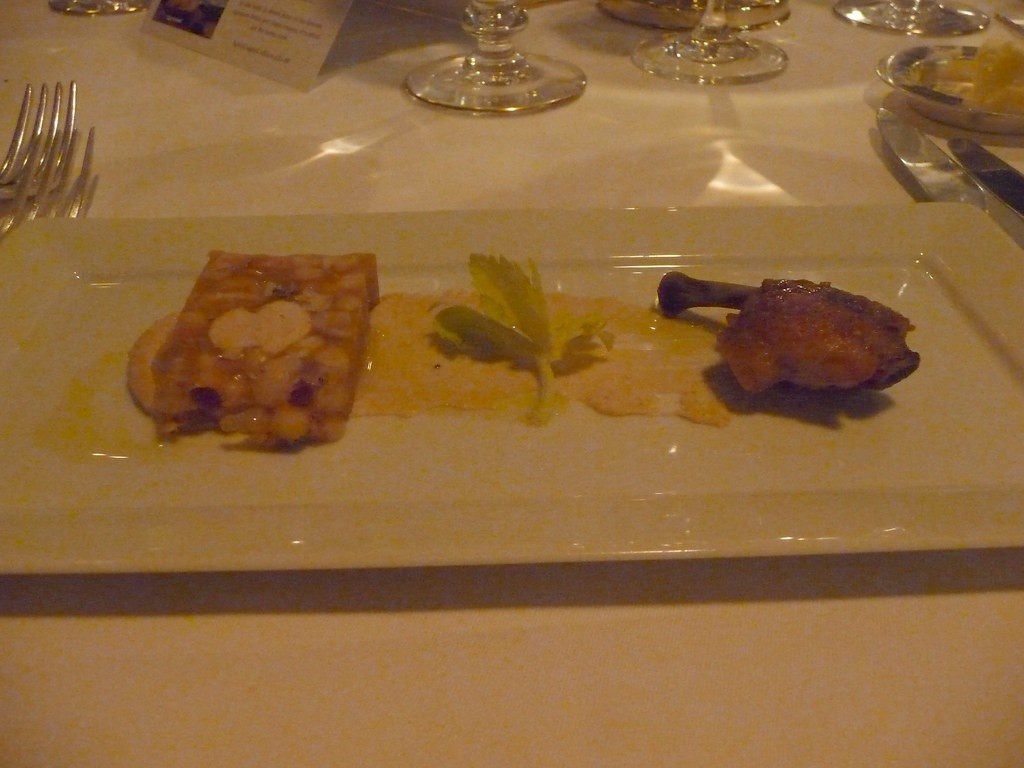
[0,80,78,207]
[0,126,96,233]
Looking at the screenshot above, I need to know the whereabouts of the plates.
[876,46,1024,136]
[0,202,1024,577]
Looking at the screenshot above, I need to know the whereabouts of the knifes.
[946,137,1024,221]
[876,107,989,217]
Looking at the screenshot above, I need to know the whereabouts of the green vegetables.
[433,251,616,422]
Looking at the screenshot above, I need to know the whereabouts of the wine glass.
[401,1,588,114]
[632,1,788,85]
[832,0,991,36]
